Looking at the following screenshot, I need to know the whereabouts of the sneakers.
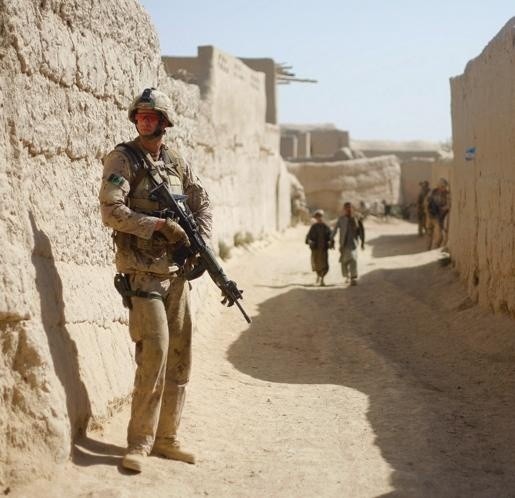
[154,438,194,463]
[123,452,145,471]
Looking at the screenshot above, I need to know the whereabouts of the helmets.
[128,88,174,126]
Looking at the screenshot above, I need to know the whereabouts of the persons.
[382,177,451,251]
[331,202,364,286]
[99,87,213,473]
[305,209,335,286]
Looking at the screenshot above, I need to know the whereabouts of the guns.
[148,182,250,323]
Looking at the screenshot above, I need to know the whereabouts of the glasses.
[134,113,158,121]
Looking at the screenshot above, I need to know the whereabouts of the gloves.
[160,217,191,247]
